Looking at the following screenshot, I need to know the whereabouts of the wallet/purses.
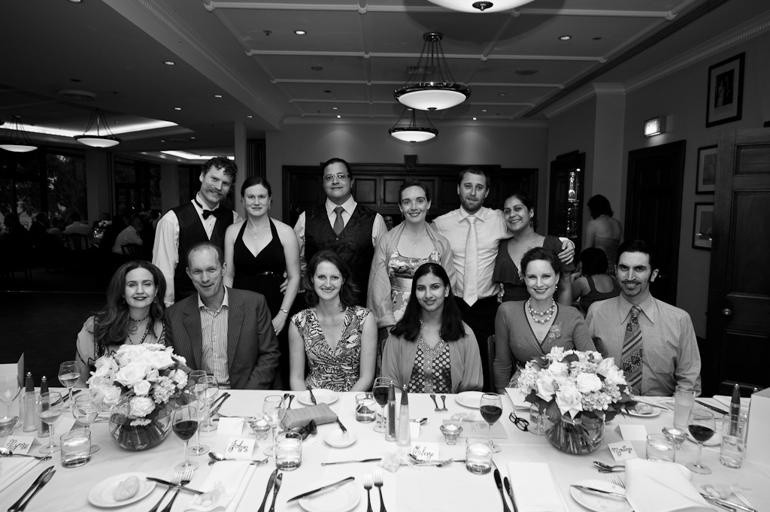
[277,402,338,433]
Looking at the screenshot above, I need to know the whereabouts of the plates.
[88,472,156,508]
[299,483,360,512]
[570,480,634,512]
[300,388,356,448]
[622,402,659,420]
[455,391,486,409]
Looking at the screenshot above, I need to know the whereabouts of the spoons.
[593,460,624,471]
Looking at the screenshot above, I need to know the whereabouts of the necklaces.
[528,298,556,324]
[129,314,150,322]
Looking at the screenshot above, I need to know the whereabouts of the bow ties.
[202,208,220,220]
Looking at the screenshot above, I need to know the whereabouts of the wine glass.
[372,377,391,433]
[645,384,757,475]
[0,360,286,469]
[479,392,502,433]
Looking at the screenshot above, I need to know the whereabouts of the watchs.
[280,309,289,314]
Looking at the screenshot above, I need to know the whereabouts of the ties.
[462,216,479,308]
[620,306,645,397]
[333,206,346,235]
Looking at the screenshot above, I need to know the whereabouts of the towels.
[173,461,256,512]
[209,417,256,466]
[507,461,570,512]
[624,459,726,512]
[0,455,43,492]
[364,439,400,473]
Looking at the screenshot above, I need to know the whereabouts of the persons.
[491,193,574,305]
[164,244,281,389]
[223,176,301,391]
[569,248,621,316]
[584,242,702,397]
[294,157,389,313]
[0,192,153,286]
[288,250,378,392]
[380,262,484,393]
[493,247,598,395]
[428,166,576,392]
[73,261,165,387]
[152,158,288,309]
[367,181,457,381]
[581,195,624,277]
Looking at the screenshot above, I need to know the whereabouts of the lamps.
[389,0,534,145]
[0,108,121,153]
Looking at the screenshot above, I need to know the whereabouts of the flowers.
[518,346,637,454]
[85,342,187,445]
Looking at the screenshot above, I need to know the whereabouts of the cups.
[440,423,493,475]
[274,429,303,470]
[353,393,376,424]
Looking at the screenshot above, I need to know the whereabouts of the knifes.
[148,477,205,497]
[257,469,354,512]
[306,385,316,405]
[321,457,382,465]
[6,465,56,512]
[493,469,518,512]
[700,492,756,512]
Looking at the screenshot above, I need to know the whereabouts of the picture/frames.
[692,50,746,251]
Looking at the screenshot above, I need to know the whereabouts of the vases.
[544,420,605,455]
[109,413,173,451]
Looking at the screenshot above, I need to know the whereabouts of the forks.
[363,472,387,512]
[148,469,193,512]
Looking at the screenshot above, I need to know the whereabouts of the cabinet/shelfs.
[350,173,439,215]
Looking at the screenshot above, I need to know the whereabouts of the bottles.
[388,380,413,448]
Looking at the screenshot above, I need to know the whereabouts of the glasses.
[322,172,351,181]
